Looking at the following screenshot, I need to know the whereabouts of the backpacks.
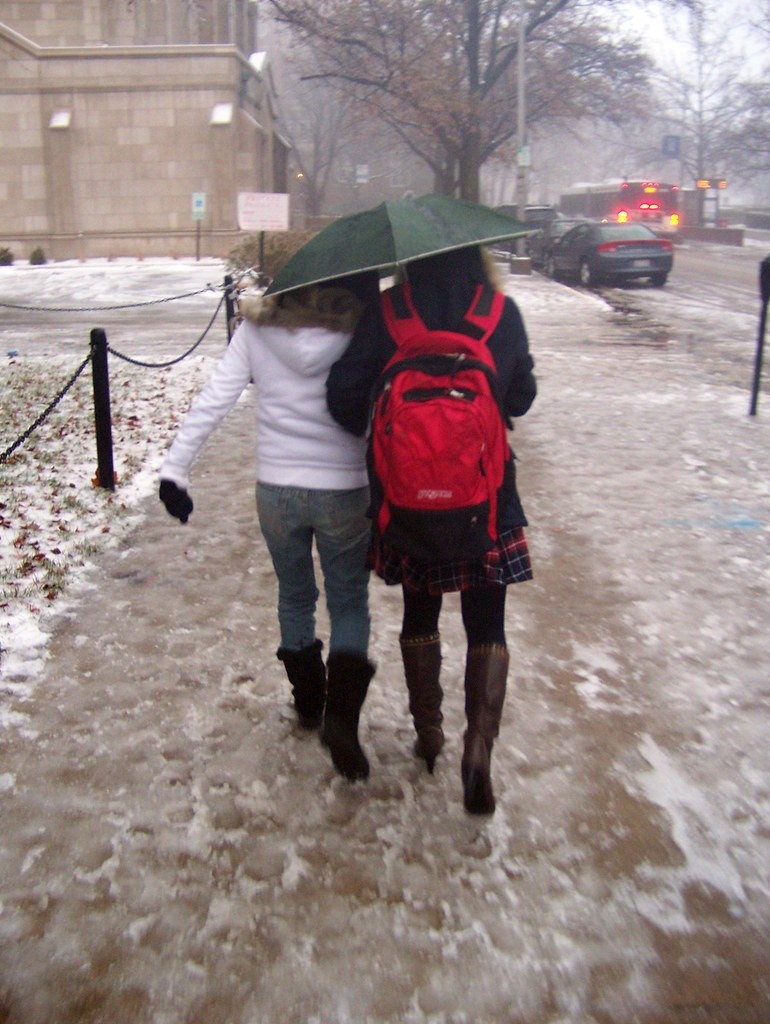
[372,282,510,510]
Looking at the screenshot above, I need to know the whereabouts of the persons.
[160,245,538,818]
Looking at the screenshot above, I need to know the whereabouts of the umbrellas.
[258,190,547,295]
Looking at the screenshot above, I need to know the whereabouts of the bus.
[557,179,682,245]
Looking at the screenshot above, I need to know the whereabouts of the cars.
[490,204,558,260]
[544,220,673,289]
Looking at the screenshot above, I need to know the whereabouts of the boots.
[398,633,445,775]
[322,651,376,780]
[276,638,327,729]
[461,641,509,814]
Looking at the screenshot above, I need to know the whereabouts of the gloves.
[159,480,193,524]
